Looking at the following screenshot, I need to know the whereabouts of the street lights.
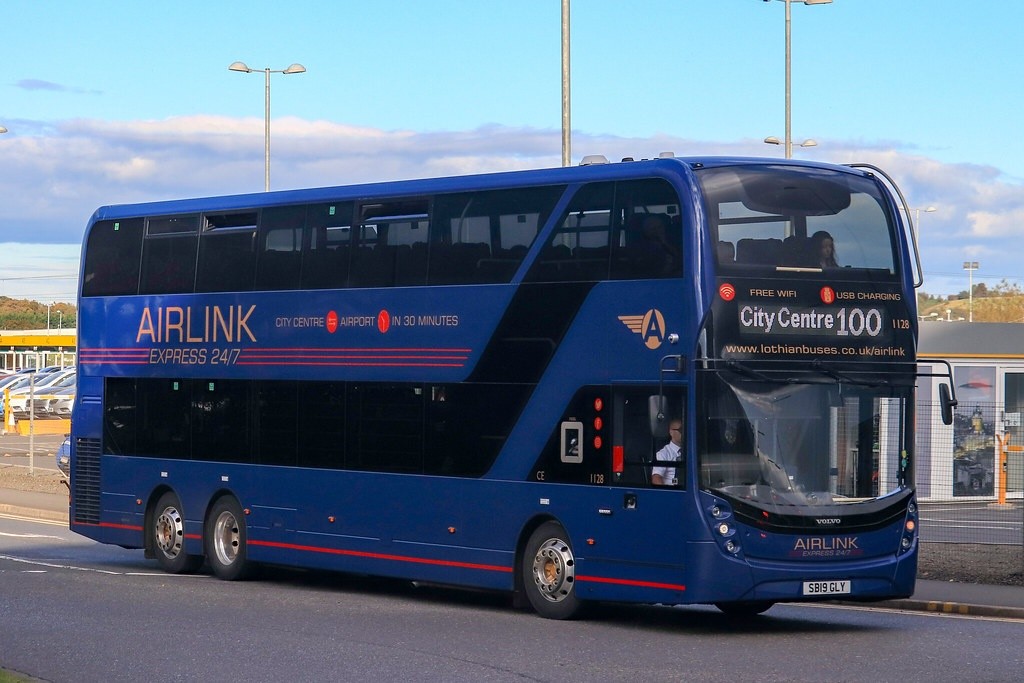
[896,204,937,324]
[756,0,838,160]
[962,261,980,322]
[56,310,64,328]
[918,311,939,322]
[937,309,966,323]
[228,61,307,195]
[763,137,820,244]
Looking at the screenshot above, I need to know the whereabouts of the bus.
[66,150,959,622]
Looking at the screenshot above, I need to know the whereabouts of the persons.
[651,420,681,485]
[807,231,839,268]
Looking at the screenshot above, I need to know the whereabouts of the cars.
[0,364,78,421]
[56,433,72,478]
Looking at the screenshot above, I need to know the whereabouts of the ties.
[674,448,682,478]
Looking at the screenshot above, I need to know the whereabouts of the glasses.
[672,426,683,433]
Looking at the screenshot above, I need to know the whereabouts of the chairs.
[264,236,811,288]
